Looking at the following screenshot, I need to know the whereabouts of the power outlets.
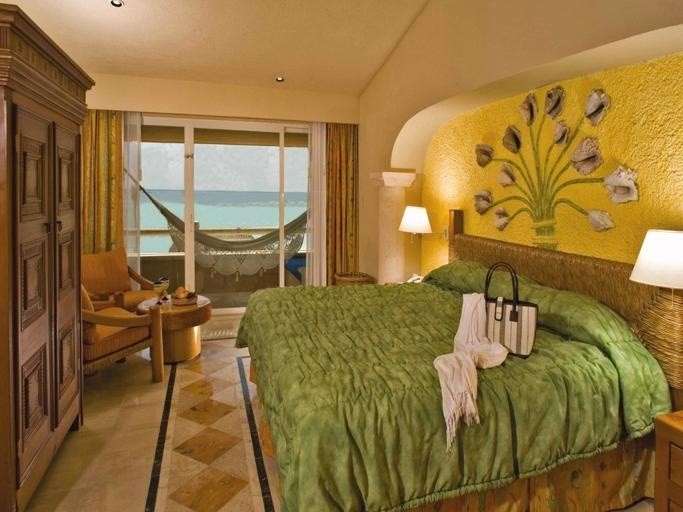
[629,228,682,294]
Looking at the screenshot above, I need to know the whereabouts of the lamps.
[398,205,433,280]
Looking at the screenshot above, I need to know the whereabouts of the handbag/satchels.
[459,262,541,360]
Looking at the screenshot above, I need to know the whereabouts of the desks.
[136,292,214,363]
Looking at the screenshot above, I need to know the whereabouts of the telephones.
[406,276,424,284]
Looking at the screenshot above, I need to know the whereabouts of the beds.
[231,206,683,509]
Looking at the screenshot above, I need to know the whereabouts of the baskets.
[171,295,198,306]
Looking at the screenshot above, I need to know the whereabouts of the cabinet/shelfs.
[0,1,100,511]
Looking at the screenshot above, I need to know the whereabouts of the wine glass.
[153,278,170,307]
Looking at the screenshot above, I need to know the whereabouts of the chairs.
[75,245,156,365]
[73,280,165,388]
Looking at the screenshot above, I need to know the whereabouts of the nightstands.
[648,411,681,511]
